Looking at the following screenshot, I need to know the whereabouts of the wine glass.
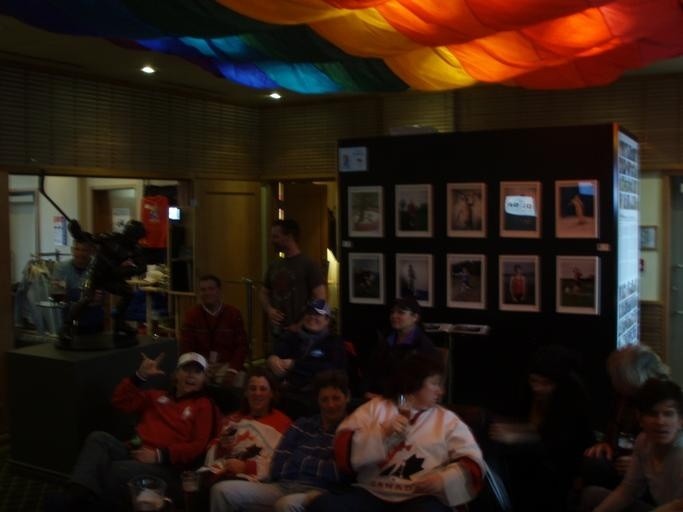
[395,392,414,443]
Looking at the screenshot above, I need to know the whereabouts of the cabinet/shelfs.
[5,334,176,470]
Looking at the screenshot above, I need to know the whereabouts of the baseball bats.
[37,187,71,224]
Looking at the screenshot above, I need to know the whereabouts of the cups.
[147,269,169,283]
[149,311,161,335]
[181,471,201,503]
[128,473,166,512]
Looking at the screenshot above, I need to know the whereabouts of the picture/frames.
[347,185,384,239]
[444,182,487,239]
[347,253,385,305]
[395,253,435,308]
[499,180,541,240]
[555,180,599,239]
[446,253,486,309]
[497,255,541,314]
[394,183,432,238]
[639,224,657,251]
[555,256,600,315]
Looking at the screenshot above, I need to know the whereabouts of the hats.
[176,352,207,373]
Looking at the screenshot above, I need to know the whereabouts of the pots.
[37,296,67,338]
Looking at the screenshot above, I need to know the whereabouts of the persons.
[464,195,477,226]
[52,219,682,511]
[569,193,587,228]
[407,197,419,227]
